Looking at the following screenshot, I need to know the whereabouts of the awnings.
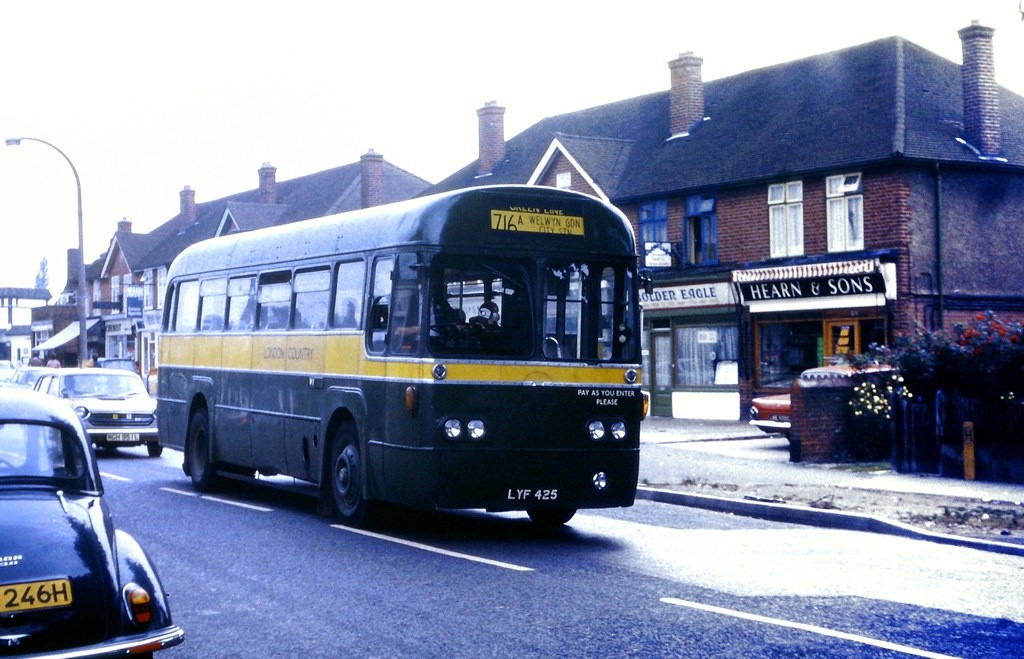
[32,319,101,350]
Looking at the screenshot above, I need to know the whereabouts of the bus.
[158,181,655,526]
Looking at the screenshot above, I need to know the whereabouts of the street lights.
[5,135,90,368]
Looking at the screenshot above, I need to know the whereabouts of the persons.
[393,286,465,352]
[479,302,499,328]
[27,353,41,367]
[45,353,61,368]
[61,376,77,393]
[92,353,101,368]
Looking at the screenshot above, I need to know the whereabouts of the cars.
[0,356,141,387]
[749,392,790,441]
[33,368,163,458]
[0,384,185,659]
